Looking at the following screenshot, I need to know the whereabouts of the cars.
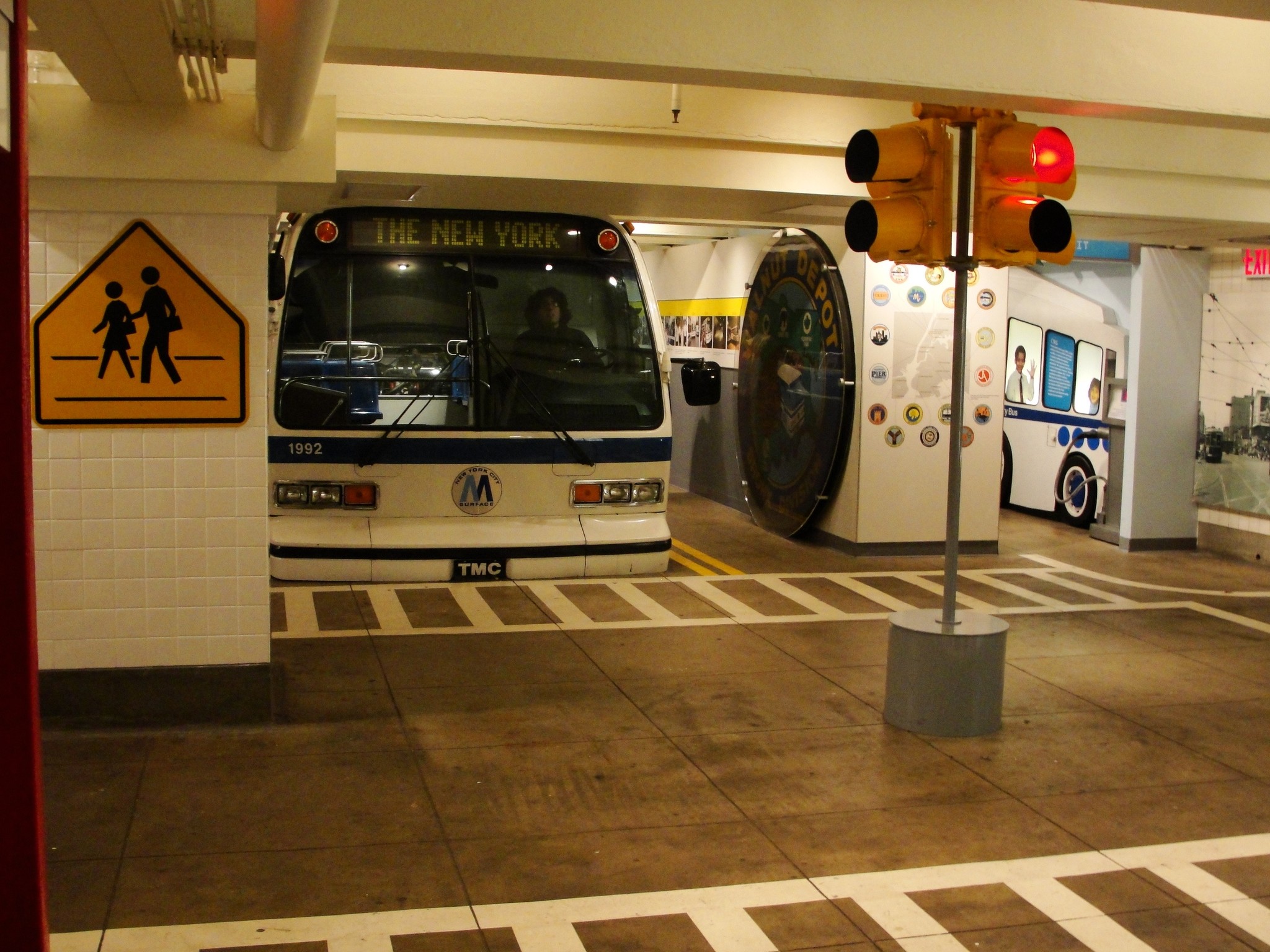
[382,352,450,396]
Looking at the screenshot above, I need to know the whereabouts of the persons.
[1088,378,1101,415]
[1006,346,1038,404]
[509,285,607,429]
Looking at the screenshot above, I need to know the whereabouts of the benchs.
[447,340,469,405]
[281,341,383,419]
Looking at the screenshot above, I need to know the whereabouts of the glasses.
[540,301,559,310]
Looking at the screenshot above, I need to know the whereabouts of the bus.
[268,208,722,583]
[1205,427,1223,462]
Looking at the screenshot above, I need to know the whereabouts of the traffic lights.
[844,121,955,269]
[974,116,1077,270]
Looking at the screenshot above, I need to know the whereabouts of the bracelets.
[1031,376,1033,379]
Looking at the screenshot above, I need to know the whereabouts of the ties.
[1020,376,1024,404]
